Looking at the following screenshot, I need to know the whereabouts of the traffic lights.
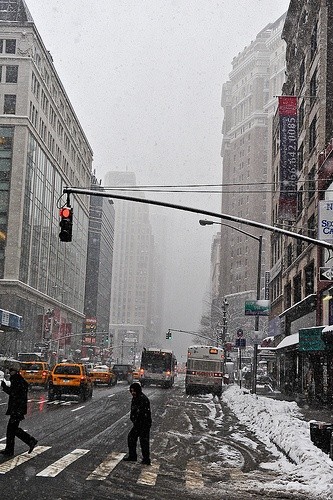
[166,332,171,340]
[106,335,108,340]
[101,335,104,342]
[59,207,73,242]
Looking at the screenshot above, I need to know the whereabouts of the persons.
[123,383,152,464]
[0,367,39,454]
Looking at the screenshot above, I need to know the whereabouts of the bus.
[139,346,177,388]
[185,345,225,396]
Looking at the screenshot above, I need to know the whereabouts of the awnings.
[276,333,299,352]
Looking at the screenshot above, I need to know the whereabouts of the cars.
[48,361,93,401]
[133,369,140,378]
[19,361,52,388]
[88,366,116,385]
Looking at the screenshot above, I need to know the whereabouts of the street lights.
[199,219,263,393]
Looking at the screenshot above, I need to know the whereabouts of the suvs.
[112,364,134,384]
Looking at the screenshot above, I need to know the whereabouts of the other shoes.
[123,457,137,461]
[0,451,14,456]
[28,440,38,454]
[139,461,151,465]
